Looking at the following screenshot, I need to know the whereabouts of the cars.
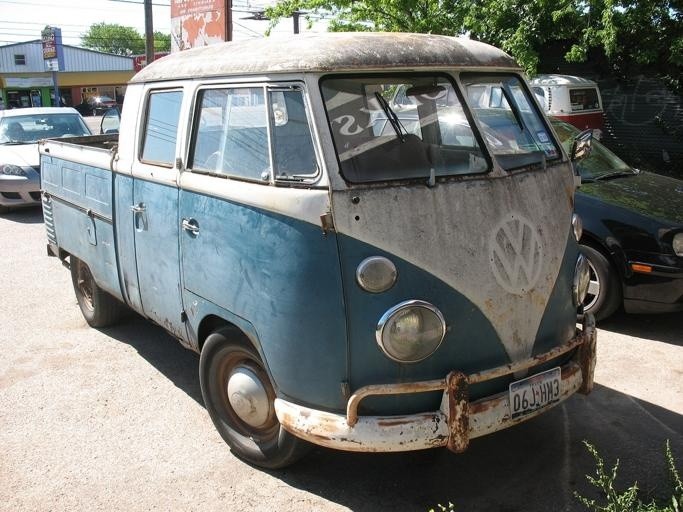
[75,95,117,117]
[0,106,93,214]
[370,105,683,323]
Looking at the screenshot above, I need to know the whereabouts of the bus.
[389,72,605,148]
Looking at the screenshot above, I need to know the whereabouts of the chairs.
[6,120,26,142]
[53,119,69,136]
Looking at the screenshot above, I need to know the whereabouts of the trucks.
[40,31,598,467]
